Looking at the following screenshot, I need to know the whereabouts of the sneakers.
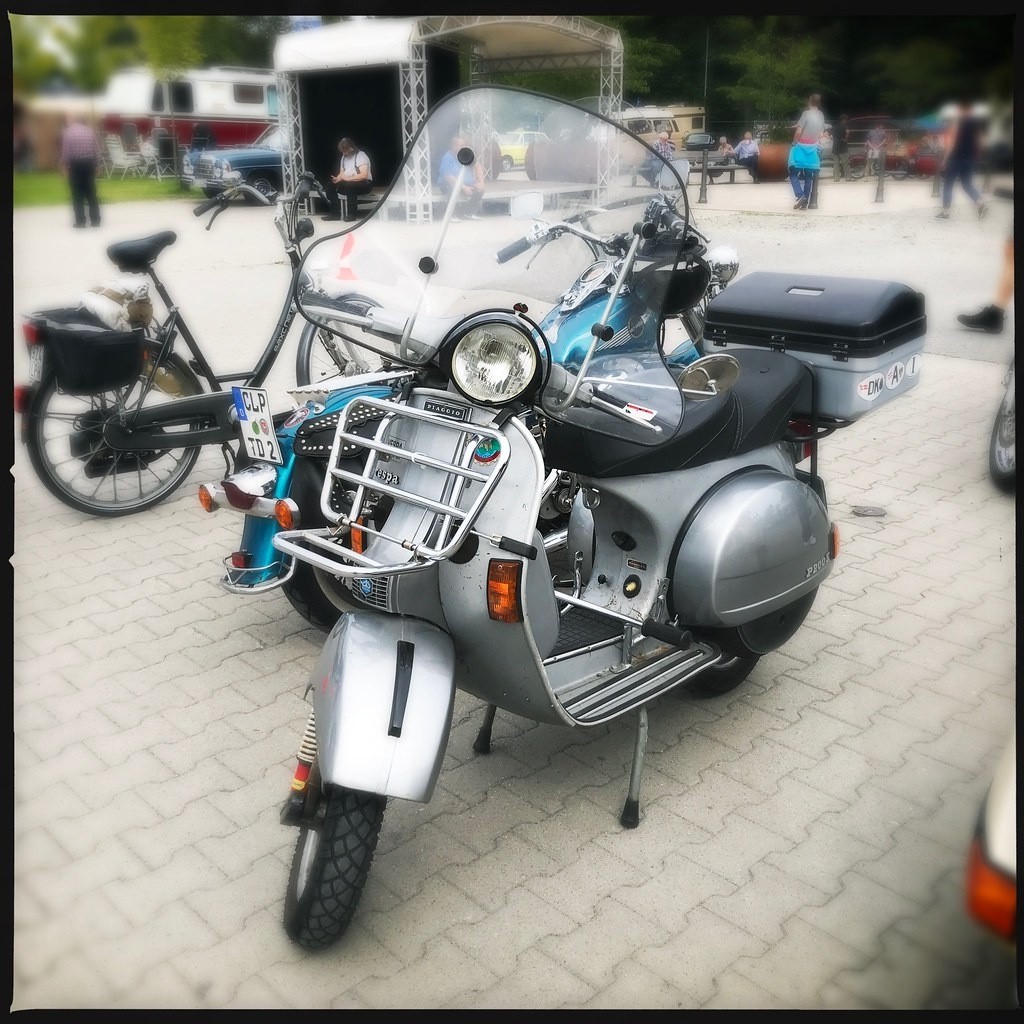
[957,306,1003,334]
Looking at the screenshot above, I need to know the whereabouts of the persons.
[707,137,733,184]
[918,98,988,220]
[438,137,485,223]
[61,110,101,228]
[321,138,373,221]
[864,120,886,182]
[729,132,760,184]
[957,222,1014,333]
[824,114,852,182]
[640,132,673,188]
[788,94,824,210]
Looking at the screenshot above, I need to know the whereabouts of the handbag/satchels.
[868,150,880,159]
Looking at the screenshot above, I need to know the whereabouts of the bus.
[585,105,708,169]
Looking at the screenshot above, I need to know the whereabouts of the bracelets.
[791,143,795,146]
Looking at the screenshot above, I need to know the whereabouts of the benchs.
[631,151,749,186]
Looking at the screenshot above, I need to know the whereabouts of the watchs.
[871,147,873,149]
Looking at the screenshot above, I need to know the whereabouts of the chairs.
[99,122,216,181]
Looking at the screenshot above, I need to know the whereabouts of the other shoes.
[321,216,340,221]
[793,196,807,209]
[754,178,760,184]
[934,212,949,219]
[343,215,356,221]
[799,205,806,210]
[847,176,857,182]
[978,204,988,219]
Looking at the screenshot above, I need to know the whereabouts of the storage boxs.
[702,269,927,424]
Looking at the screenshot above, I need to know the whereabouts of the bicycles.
[847,139,954,179]
[9,163,376,524]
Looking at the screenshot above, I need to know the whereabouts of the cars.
[494,126,549,173]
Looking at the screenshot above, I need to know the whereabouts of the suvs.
[179,122,288,204]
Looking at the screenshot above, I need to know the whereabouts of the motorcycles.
[197,83,932,956]
